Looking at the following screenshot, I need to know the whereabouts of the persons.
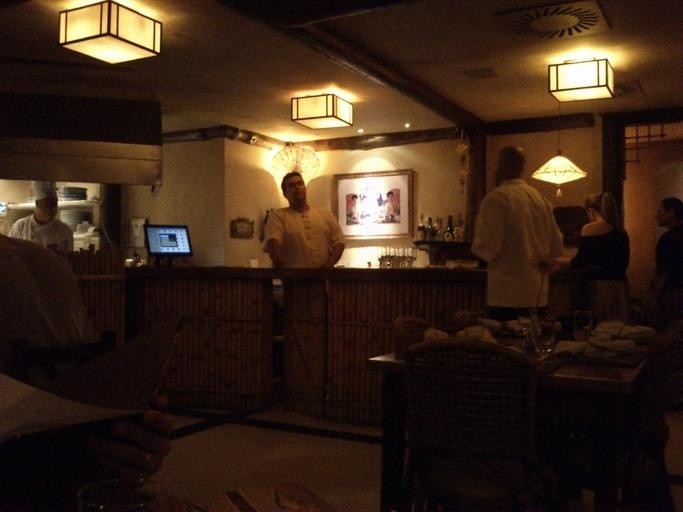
[6,180,73,256]
[0,235,171,512]
[642,197,683,329]
[261,173,347,269]
[556,190,630,325]
[462,146,565,320]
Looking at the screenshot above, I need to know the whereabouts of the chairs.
[399,337,547,508]
[617,331,679,510]
[390,316,433,357]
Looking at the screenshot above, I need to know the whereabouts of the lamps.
[57,0,163,69]
[292,94,357,131]
[530,99,588,199]
[543,59,619,107]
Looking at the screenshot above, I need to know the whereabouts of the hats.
[30,180,58,201]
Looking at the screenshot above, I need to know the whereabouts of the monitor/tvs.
[143,224,193,266]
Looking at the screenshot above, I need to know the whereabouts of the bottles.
[551,322,562,343]
[525,308,545,364]
[416,212,464,241]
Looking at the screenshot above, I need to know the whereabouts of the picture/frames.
[328,169,415,245]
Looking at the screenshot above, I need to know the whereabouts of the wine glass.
[376,246,419,268]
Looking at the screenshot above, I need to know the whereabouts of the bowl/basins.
[61,188,86,201]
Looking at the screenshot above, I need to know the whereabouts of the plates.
[59,209,90,232]
[588,336,608,347]
